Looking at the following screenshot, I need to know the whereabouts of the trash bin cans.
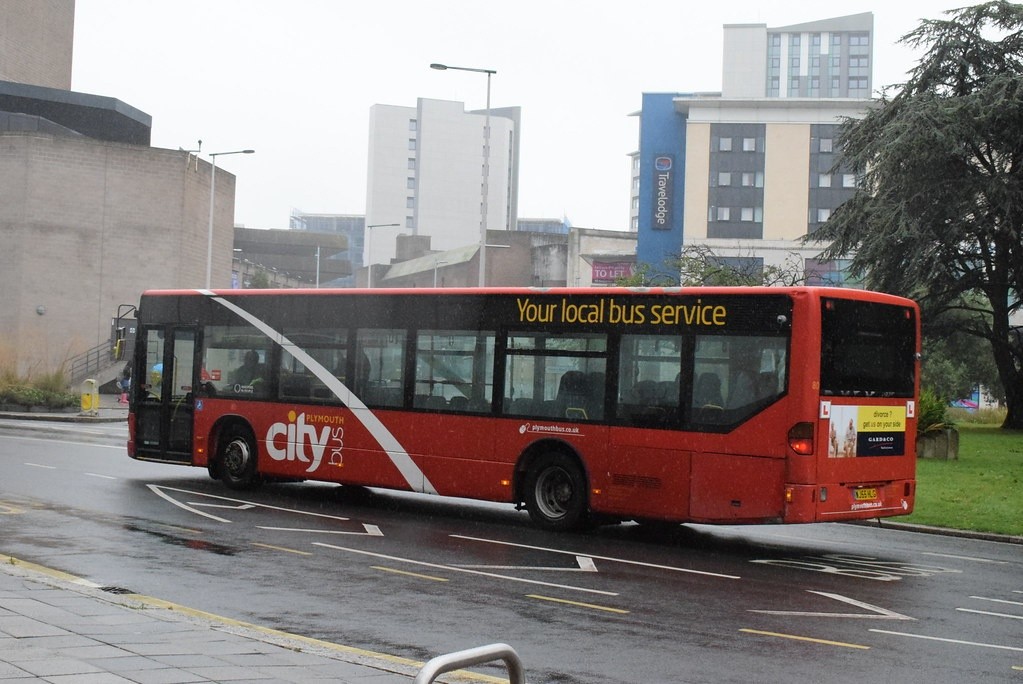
[80,379,99,416]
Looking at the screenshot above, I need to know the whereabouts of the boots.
[122,394,129,403]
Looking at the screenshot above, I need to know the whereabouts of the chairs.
[284,371,779,425]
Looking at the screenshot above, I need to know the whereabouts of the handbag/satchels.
[122,379,129,387]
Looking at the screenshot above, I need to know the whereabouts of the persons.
[540,371,781,418]
[334,341,370,401]
[235,351,265,386]
[121,361,132,402]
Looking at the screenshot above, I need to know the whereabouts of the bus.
[113,285,926,535]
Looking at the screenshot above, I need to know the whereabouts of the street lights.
[429,63,511,287]
[434,259,448,286]
[206,149,256,289]
[366,223,401,288]
